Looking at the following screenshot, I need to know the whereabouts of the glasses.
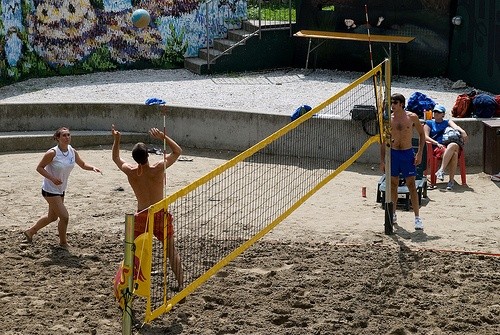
[390,101,399,105]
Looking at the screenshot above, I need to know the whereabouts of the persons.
[111,128,184,291]
[423,104,467,189]
[24,127,103,246]
[380,93,425,229]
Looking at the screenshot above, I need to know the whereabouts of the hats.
[431,105,446,113]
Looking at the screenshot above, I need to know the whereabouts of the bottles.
[362,186,366,197]
[423,109,426,120]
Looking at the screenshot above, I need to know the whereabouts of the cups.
[426,111,432,120]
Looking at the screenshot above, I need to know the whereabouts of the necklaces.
[59,146,69,156]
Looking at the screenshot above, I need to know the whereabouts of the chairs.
[424,140,466,188]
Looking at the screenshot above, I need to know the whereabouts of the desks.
[294,30,417,81]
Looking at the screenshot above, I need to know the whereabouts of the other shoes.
[435,169,445,181]
[414,219,423,230]
[383,213,397,226]
[446,180,455,190]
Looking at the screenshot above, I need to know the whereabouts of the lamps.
[451,16,463,26]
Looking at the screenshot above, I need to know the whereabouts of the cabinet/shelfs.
[482,120,500,175]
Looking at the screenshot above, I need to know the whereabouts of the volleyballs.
[130,8,151,28]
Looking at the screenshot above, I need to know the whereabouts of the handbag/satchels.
[349,104,376,121]
